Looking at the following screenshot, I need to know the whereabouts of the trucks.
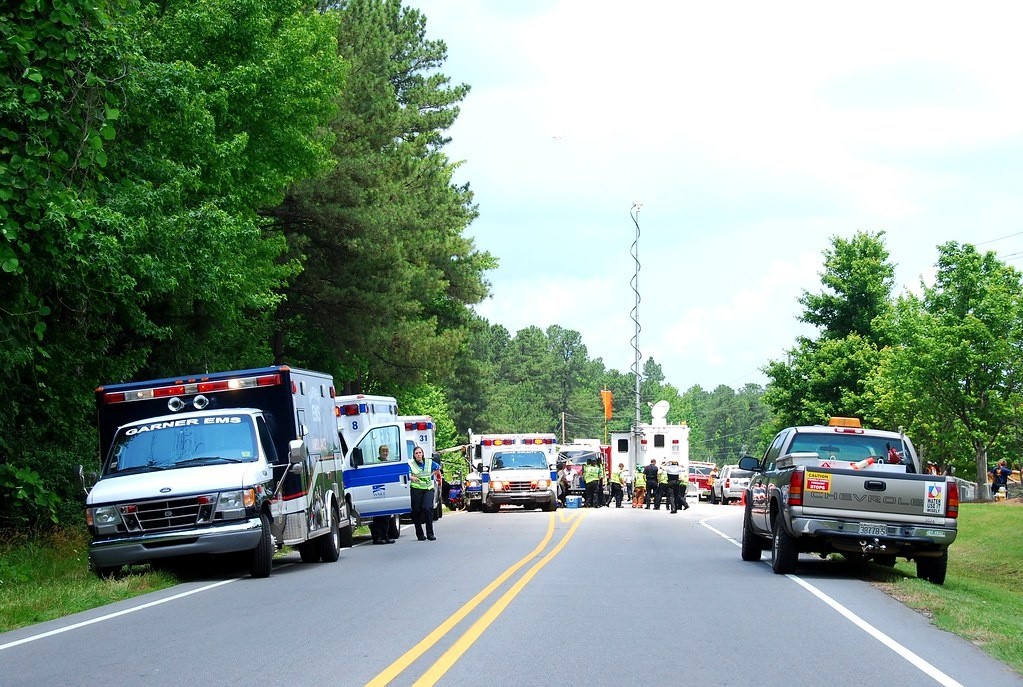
[689,461,716,501]
[465,435,492,512]
[78,366,350,577]
[481,433,557,513]
[558,443,611,506]
[336,394,412,548]
[397,416,443,523]
[631,400,690,504]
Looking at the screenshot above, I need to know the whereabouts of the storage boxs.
[565,495,583,508]
[775,452,820,467]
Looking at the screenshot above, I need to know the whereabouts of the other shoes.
[386,539,395,544]
[671,511,677,513]
[617,506,623,508]
[684,506,688,510]
[606,503,609,507]
[653,507,660,510]
[418,536,426,540]
[428,536,436,540]
[645,507,650,509]
[373,538,385,544]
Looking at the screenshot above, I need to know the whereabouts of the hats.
[565,460,573,466]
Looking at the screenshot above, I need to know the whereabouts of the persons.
[708,467,719,503]
[987,459,1023,502]
[368,443,396,545]
[557,457,605,508]
[407,446,439,541]
[606,459,690,514]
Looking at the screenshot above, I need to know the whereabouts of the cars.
[710,465,756,505]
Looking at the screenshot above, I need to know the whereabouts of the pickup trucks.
[738,425,959,585]
